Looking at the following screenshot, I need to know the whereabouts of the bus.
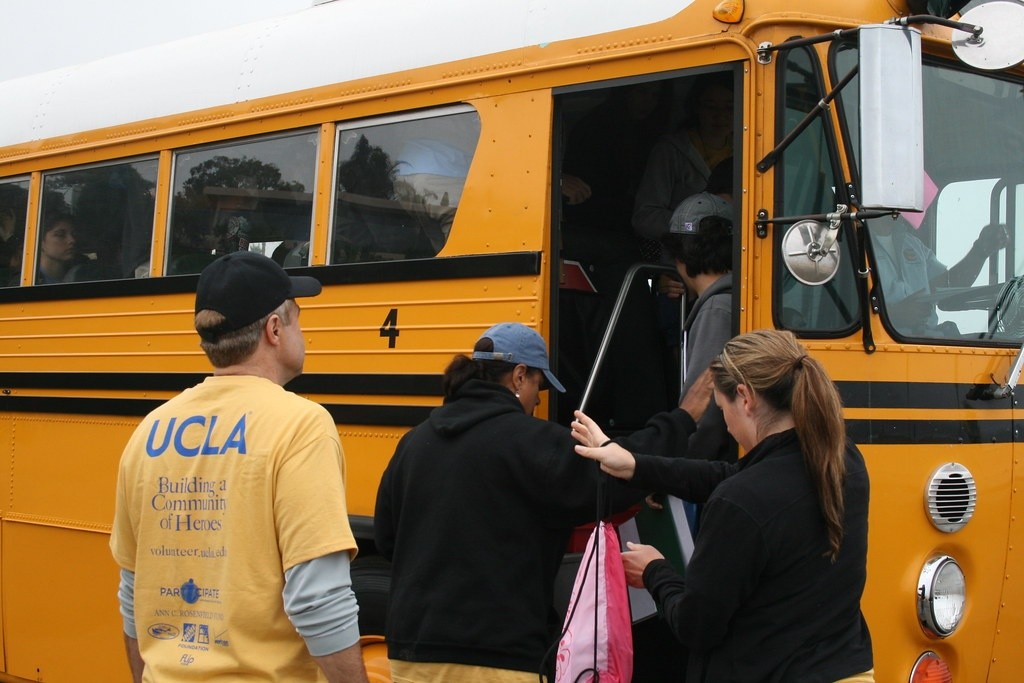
[0,2,1024,682]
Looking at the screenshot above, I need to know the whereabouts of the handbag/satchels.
[537,435,636,683]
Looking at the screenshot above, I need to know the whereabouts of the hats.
[195,251,321,341]
[471,321,567,394]
[668,189,734,235]
[41,200,77,225]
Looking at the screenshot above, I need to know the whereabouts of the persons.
[370,324,714,683]
[572,328,875,683]
[0,208,24,286]
[34,204,91,284]
[108,253,370,683]
[663,191,734,433]
[816,209,1007,331]
[559,70,735,299]
